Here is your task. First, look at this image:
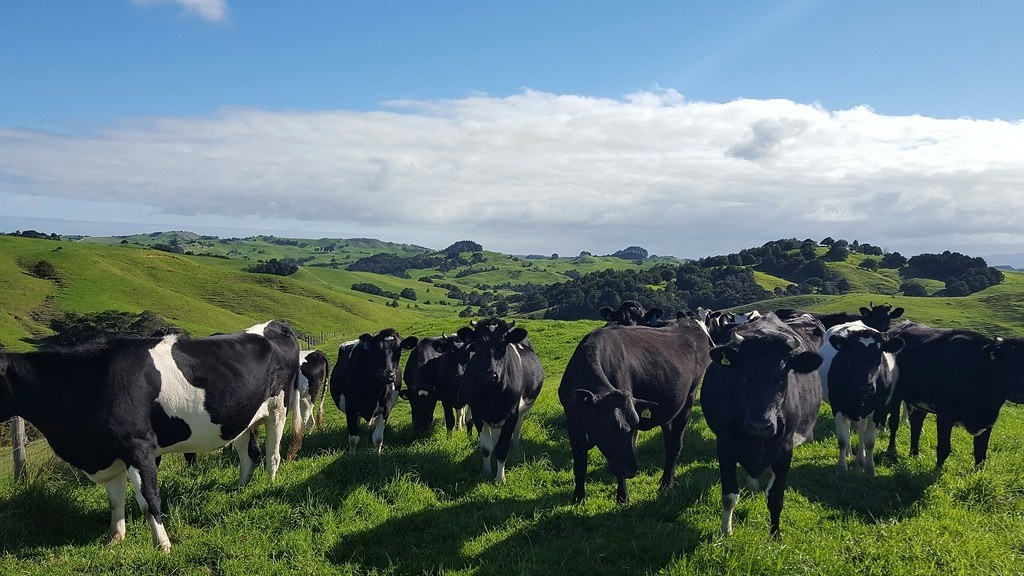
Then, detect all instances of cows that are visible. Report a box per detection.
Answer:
[555,297,1024,542]
[0,304,545,555]
